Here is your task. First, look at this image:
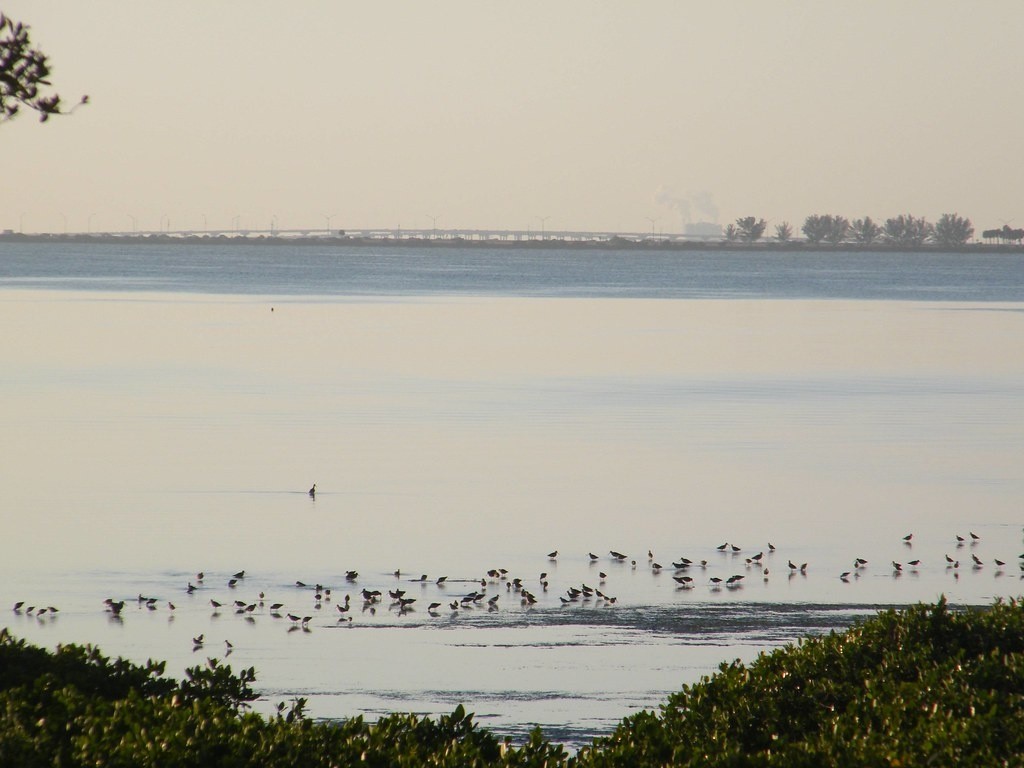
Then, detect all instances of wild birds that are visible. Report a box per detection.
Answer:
[308,484,317,493]
[0,541,808,650]
[839,531,1006,578]
